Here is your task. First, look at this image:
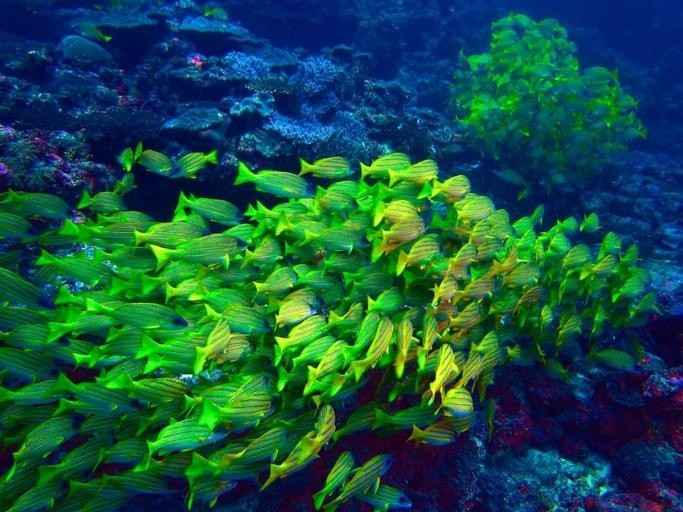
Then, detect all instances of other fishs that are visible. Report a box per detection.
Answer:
[1,141,664,512]
